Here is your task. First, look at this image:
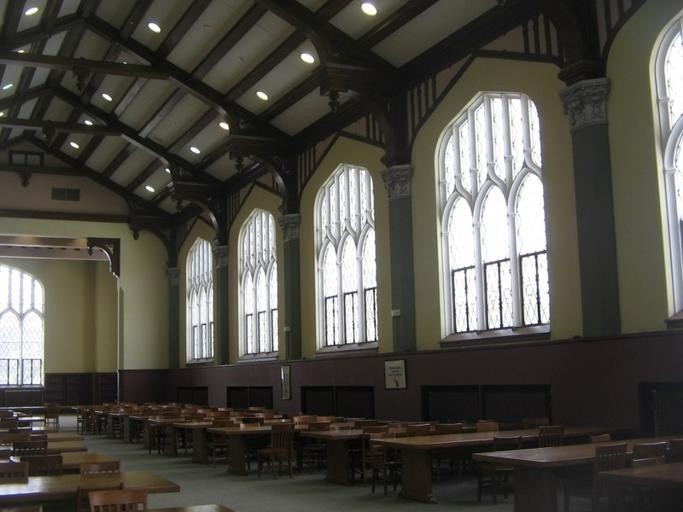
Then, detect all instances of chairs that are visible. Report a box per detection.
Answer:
[0,396,682,512]
